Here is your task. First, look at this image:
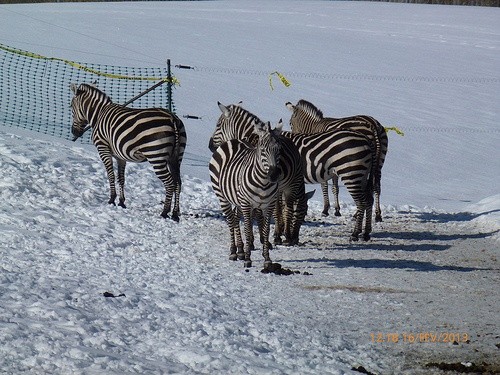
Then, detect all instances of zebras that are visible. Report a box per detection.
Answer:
[207,98,389,270]
[67,81,187,224]
